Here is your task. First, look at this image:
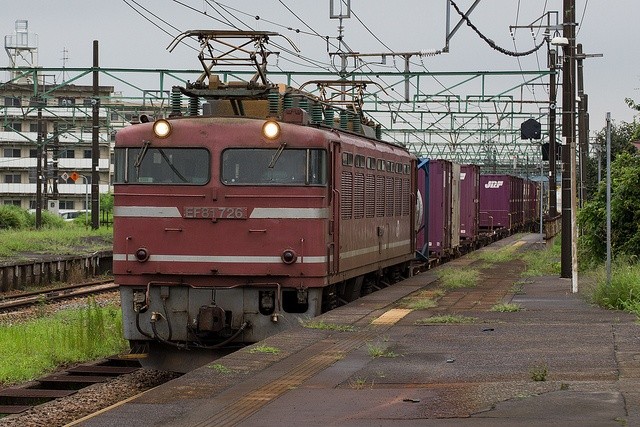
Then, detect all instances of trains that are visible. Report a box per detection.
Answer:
[113,27,540,372]
